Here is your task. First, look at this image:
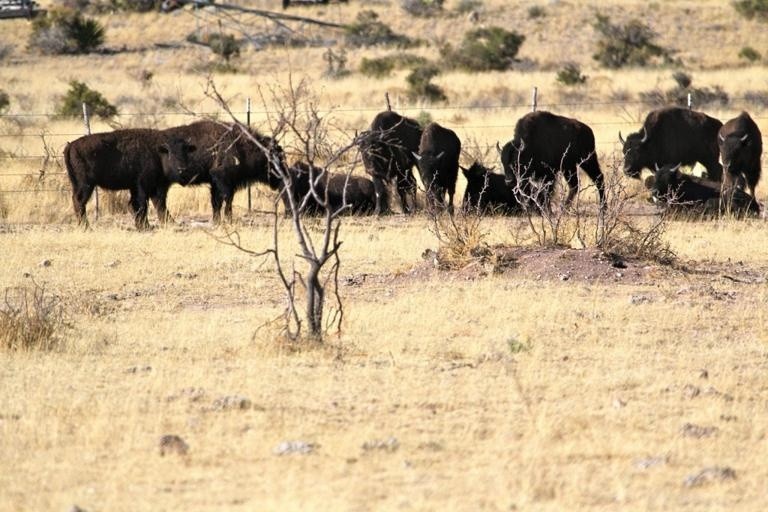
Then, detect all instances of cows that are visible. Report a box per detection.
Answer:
[459,159,519,216]
[354,111,461,216]
[64,120,287,233]
[494,110,608,216]
[278,157,377,217]
[616,108,763,222]
